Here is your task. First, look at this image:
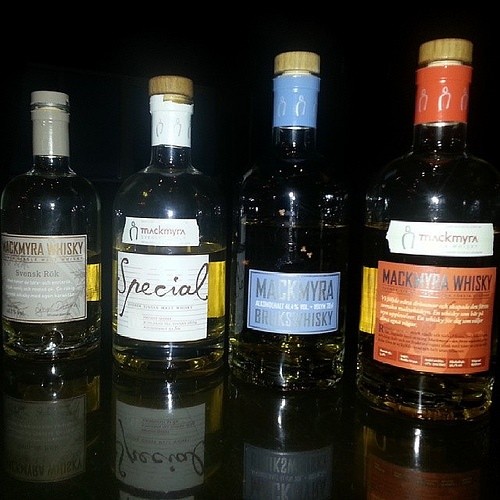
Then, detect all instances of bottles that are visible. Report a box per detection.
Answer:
[242,391,344,500]
[112,76,228,379]
[228,51,349,391]
[2,91,103,362]
[1,362,101,500]
[357,39,500,420]
[362,407,487,500]
[111,377,223,500]
